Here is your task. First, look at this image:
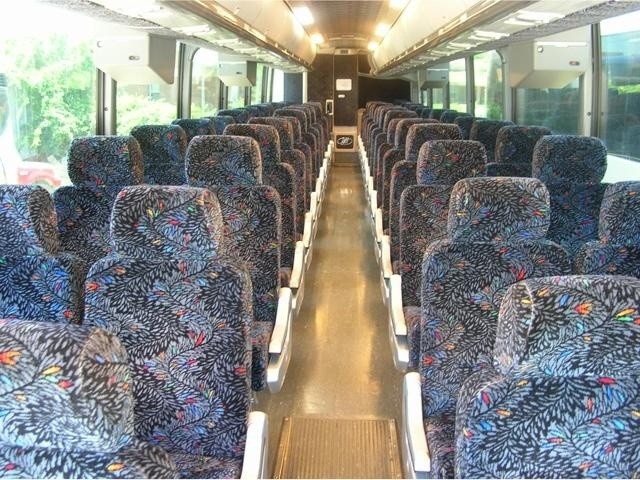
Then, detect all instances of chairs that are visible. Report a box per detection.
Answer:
[359,100,556,301]
[525,132,621,257]
[582,177,638,276]
[384,138,502,375]
[451,270,640,476]
[393,173,574,476]
[0,181,84,330]
[179,132,296,399]
[43,132,148,266]
[122,98,333,317]
[81,184,273,477]
[0,314,179,476]
